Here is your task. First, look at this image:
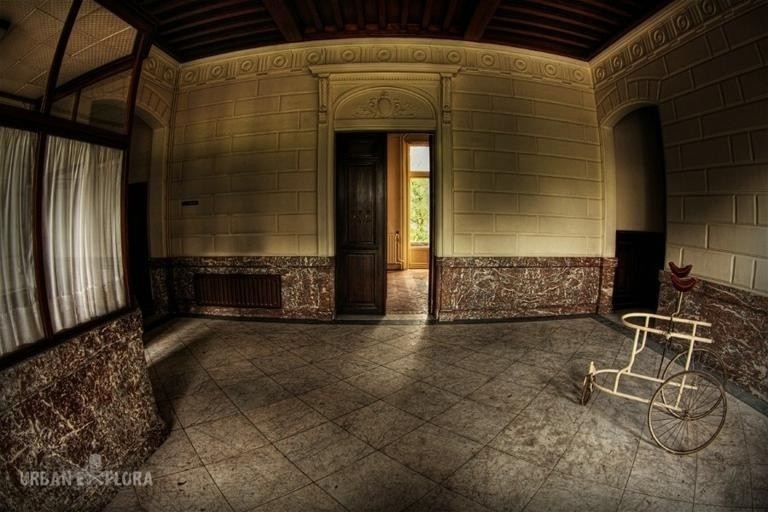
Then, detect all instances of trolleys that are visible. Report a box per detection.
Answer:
[573,261,740,461]
